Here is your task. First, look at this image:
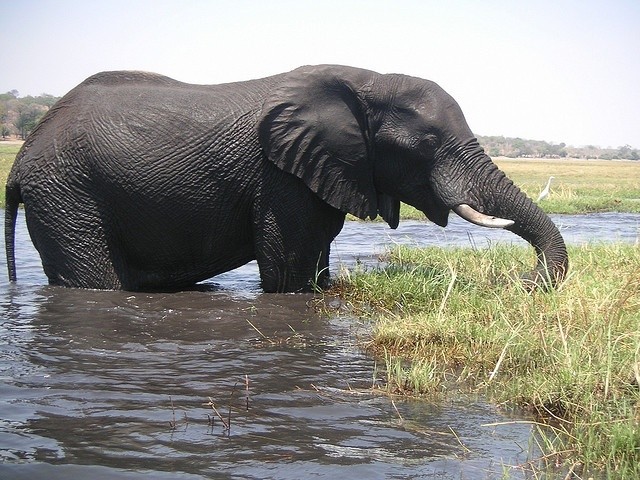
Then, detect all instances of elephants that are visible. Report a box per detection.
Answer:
[4,64,570,294]
